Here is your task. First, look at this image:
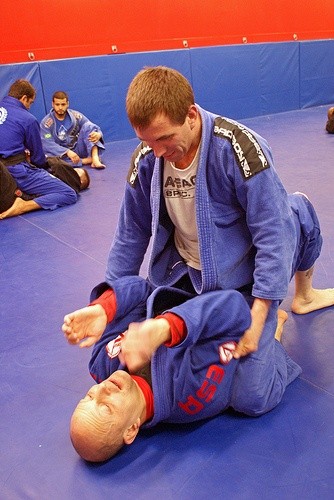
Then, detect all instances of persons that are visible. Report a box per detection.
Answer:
[39,91,106,169]
[0,79,78,219]
[102,65,303,418]
[60,192,334,463]
[0,156,90,212]
[326,106,334,133]
